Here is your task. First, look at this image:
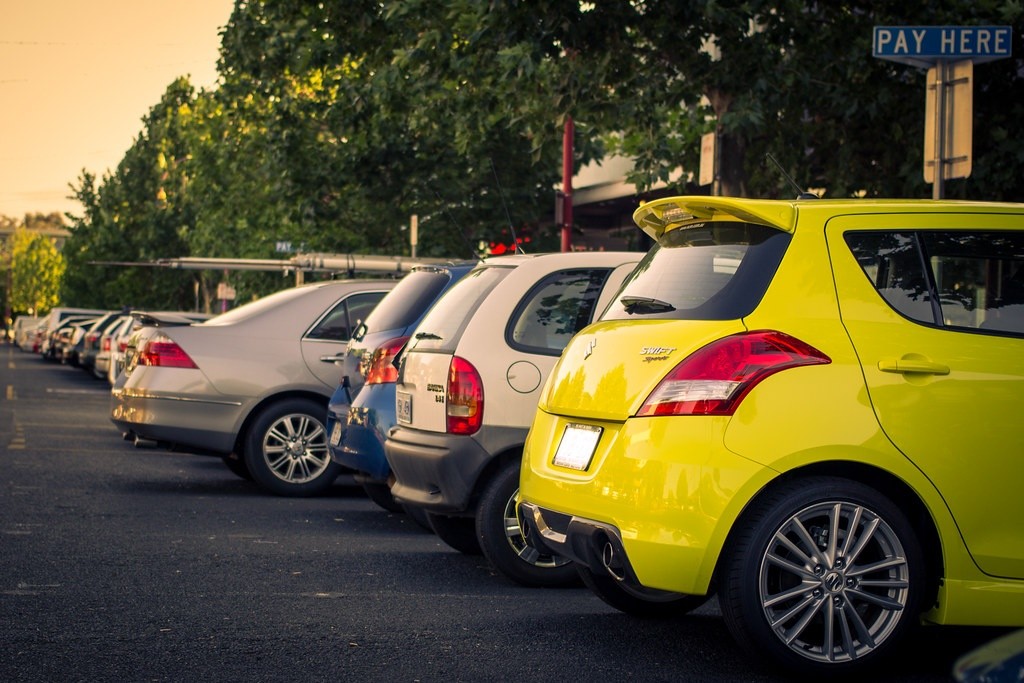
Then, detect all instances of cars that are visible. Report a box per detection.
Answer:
[513,197,1024,683]
[10,248,738,584]
